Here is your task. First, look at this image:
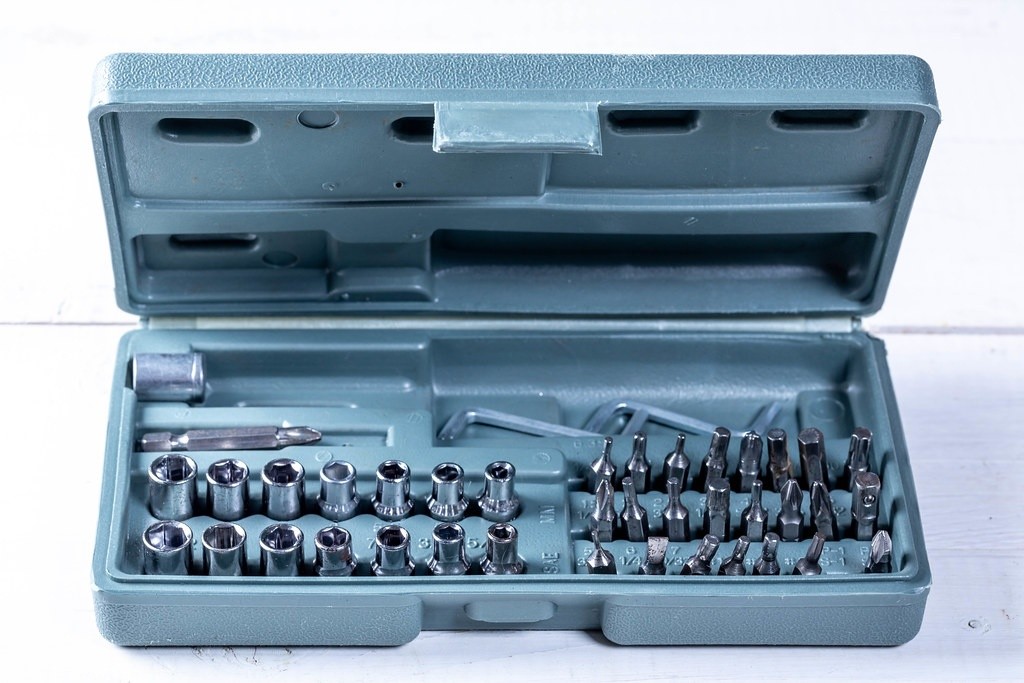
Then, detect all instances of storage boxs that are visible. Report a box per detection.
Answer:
[88,50,944,646]
